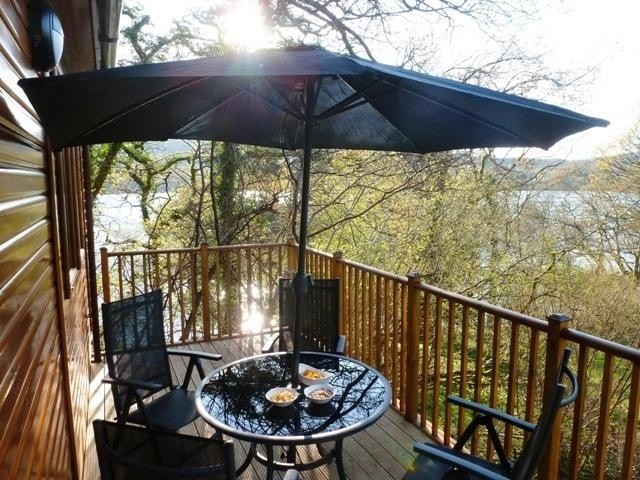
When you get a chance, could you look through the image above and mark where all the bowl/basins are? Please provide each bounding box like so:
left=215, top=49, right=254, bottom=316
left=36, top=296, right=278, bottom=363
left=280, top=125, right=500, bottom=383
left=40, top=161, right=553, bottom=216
left=303, top=384, right=337, bottom=405
left=298, top=363, right=336, bottom=387
left=265, top=387, right=299, bottom=408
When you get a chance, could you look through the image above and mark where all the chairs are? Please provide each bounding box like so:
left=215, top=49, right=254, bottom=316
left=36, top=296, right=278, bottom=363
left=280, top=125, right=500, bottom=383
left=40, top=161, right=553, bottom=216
left=401, top=347, right=579, bottom=480
left=93, top=288, right=239, bottom=479
left=262, top=275, right=346, bottom=357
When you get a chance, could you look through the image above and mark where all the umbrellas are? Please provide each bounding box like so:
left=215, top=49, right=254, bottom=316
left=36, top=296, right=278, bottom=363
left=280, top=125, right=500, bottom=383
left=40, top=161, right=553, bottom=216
left=17, top=42, right=609, bottom=465
